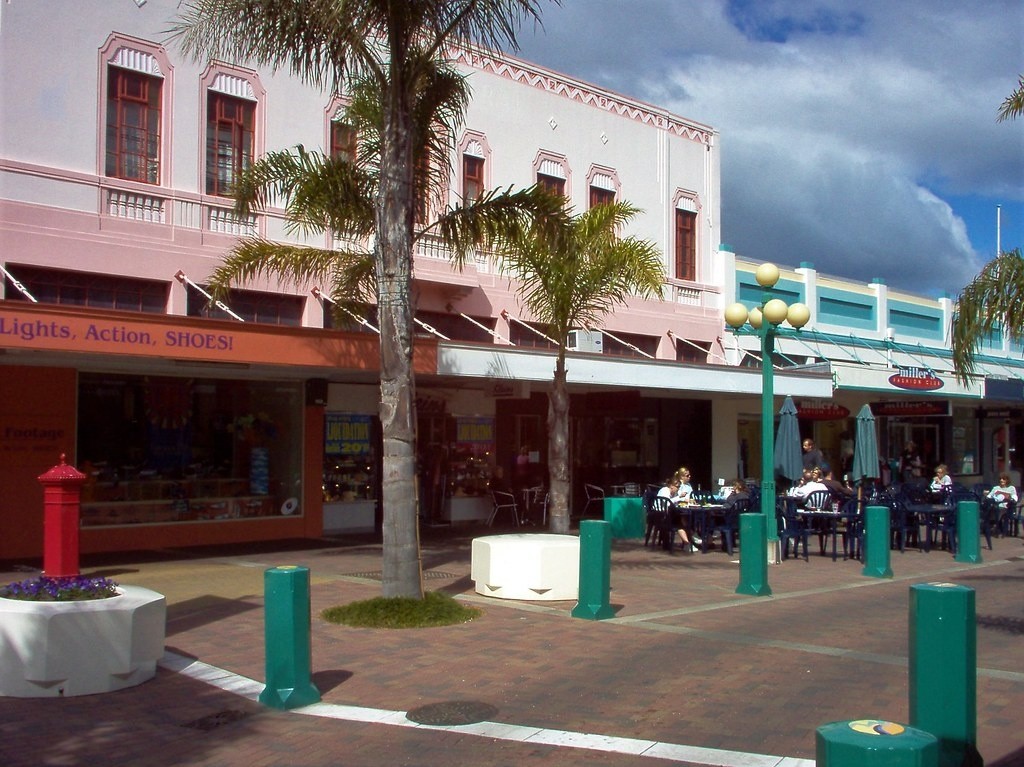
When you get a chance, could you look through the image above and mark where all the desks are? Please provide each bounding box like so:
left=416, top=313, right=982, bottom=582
left=907, top=502, right=954, bottom=552
left=781, top=494, right=804, bottom=535
left=794, top=507, right=854, bottom=562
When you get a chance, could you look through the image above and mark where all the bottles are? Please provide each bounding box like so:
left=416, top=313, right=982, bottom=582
left=697, top=484, right=703, bottom=504
left=703, top=495, right=707, bottom=505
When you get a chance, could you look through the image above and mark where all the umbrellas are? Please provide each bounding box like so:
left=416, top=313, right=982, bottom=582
left=852, top=403, right=880, bottom=515
left=774, top=394, right=803, bottom=486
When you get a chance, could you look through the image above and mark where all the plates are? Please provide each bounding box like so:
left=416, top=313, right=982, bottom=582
left=711, top=505, right=723, bottom=507
left=689, top=504, right=701, bottom=506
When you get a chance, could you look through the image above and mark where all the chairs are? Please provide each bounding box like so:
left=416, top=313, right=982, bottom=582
left=473, top=456, right=1024, bottom=566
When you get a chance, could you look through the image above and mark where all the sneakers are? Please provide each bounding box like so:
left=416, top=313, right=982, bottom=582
left=691, top=533, right=702, bottom=544
left=684, top=545, right=699, bottom=552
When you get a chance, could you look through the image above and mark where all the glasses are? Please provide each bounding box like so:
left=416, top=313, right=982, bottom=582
left=675, top=485, right=681, bottom=488
left=684, top=473, right=691, bottom=477
left=813, top=472, right=818, bottom=474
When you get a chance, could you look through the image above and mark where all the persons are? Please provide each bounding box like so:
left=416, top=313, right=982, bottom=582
left=674, top=467, right=693, bottom=503
left=930, top=464, right=952, bottom=521
left=898, top=440, right=920, bottom=480
left=490, top=466, right=525, bottom=523
left=985, top=472, right=1018, bottom=538
left=653, top=477, right=702, bottom=551
left=785, top=440, right=853, bottom=529
left=723, top=479, right=753, bottom=510
left=904, top=469, right=932, bottom=494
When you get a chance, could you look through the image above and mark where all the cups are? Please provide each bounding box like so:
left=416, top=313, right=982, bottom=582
left=832, top=503, right=839, bottom=514
left=690, top=499, right=696, bottom=505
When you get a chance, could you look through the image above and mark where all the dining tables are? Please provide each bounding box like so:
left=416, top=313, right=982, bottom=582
left=677, top=498, right=727, bottom=556
